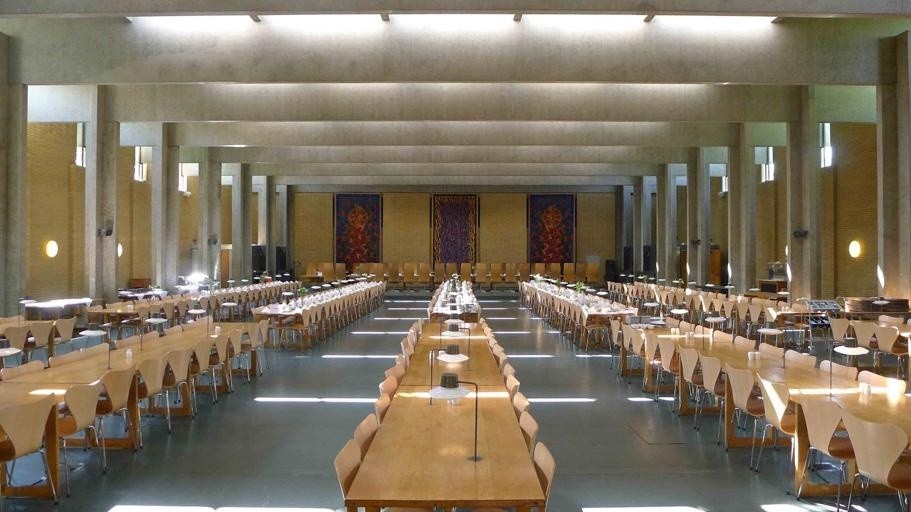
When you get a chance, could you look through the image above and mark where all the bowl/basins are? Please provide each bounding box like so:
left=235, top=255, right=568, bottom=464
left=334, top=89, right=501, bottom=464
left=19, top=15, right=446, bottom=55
left=632, top=323, right=654, bottom=330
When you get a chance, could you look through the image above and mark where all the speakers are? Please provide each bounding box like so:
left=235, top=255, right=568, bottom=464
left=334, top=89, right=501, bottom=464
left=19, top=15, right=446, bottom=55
left=605, top=259, right=617, bottom=281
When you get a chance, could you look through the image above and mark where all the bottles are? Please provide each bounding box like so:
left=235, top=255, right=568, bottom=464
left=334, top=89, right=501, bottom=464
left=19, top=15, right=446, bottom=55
left=747, top=352, right=760, bottom=361
left=215, top=326, right=220, bottom=333
left=670, top=327, right=679, bottom=334
left=781, top=305, right=791, bottom=312
left=685, top=332, right=694, bottom=340
left=865, top=385, right=872, bottom=397
left=127, top=349, right=132, bottom=358
left=738, top=293, right=744, bottom=304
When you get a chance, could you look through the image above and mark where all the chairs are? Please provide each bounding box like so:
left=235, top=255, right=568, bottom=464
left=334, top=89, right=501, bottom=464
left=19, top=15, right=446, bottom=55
left=2, top=262, right=388, bottom=510
left=333, top=263, right=558, bottom=511
left=518, top=263, right=911, bottom=512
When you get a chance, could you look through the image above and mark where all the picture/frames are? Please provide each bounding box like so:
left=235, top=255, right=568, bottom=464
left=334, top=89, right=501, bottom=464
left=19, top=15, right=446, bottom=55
left=429, top=192, right=478, bottom=267
left=528, top=191, right=577, bottom=265
left=333, top=191, right=382, bottom=268
